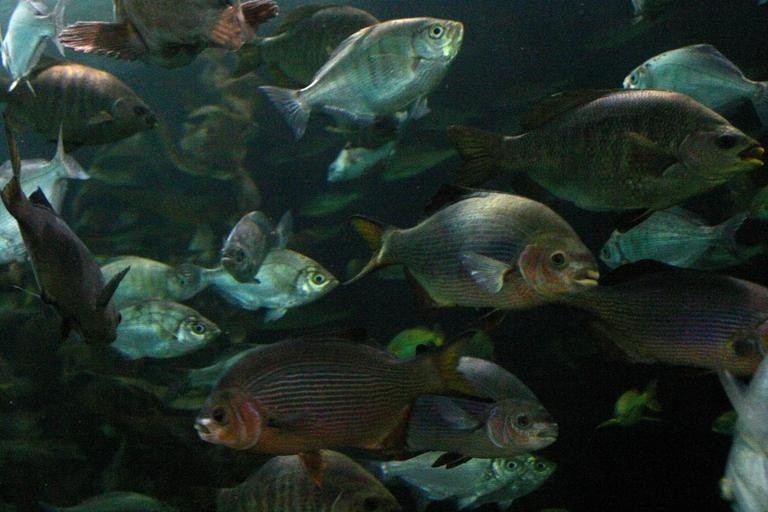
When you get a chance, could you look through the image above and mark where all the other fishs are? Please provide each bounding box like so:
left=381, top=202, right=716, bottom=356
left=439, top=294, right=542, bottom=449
left=0, top=1, right=560, bottom=512
